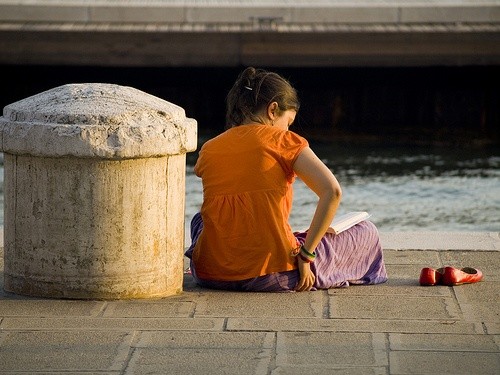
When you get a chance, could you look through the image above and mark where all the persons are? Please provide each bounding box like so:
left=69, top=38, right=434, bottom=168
left=184, top=66, right=389, bottom=293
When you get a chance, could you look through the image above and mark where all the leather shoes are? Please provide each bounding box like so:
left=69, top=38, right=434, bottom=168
left=419, top=265, right=483, bottom=287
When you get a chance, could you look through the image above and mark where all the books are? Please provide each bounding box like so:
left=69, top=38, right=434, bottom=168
left=293, top=211, right=372, bottom=235
